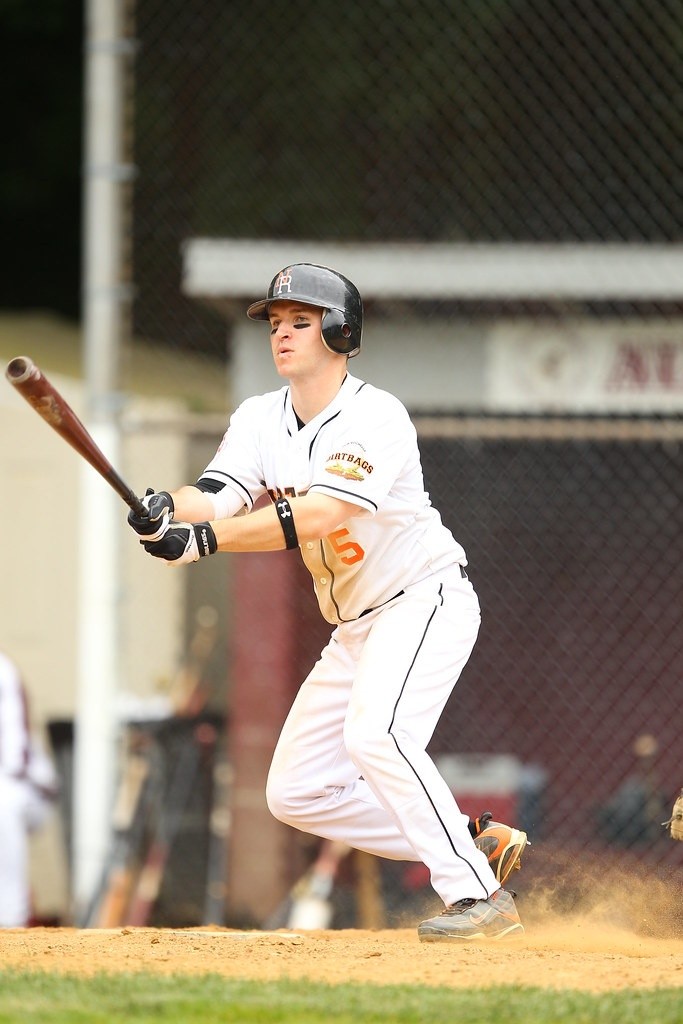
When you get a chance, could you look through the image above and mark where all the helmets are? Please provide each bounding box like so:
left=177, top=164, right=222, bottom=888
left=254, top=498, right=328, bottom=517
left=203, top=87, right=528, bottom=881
left=246, top=263, right=363, bottom=358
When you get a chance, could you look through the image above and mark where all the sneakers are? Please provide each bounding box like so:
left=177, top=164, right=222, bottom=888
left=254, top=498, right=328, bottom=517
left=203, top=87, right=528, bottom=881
left=418, top=887, right=525, bottom=943
left=472, top=810, right=532, bottom=885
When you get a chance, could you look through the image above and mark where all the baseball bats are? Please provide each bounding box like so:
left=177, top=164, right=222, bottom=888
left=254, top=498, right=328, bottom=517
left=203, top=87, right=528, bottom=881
left=1, top=353, right=151, bottom=519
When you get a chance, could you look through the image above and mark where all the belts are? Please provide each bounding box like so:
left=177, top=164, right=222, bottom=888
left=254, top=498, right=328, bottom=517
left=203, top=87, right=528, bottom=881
left=354, top=561, right=468, bottom=621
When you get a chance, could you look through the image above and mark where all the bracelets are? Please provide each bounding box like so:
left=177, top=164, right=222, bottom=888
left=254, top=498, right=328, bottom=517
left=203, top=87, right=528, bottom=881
left=275, top=498, right=298, bottom=550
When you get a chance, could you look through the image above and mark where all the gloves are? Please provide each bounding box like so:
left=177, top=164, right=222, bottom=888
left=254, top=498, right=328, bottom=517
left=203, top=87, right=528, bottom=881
left=127, top=488, right=174, bottom=541
left=140, top=519, right=218, bottom=568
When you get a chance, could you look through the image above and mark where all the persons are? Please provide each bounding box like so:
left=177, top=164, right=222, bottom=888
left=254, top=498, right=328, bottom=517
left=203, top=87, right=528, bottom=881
left=128, top=263, right=527, bottom=943
